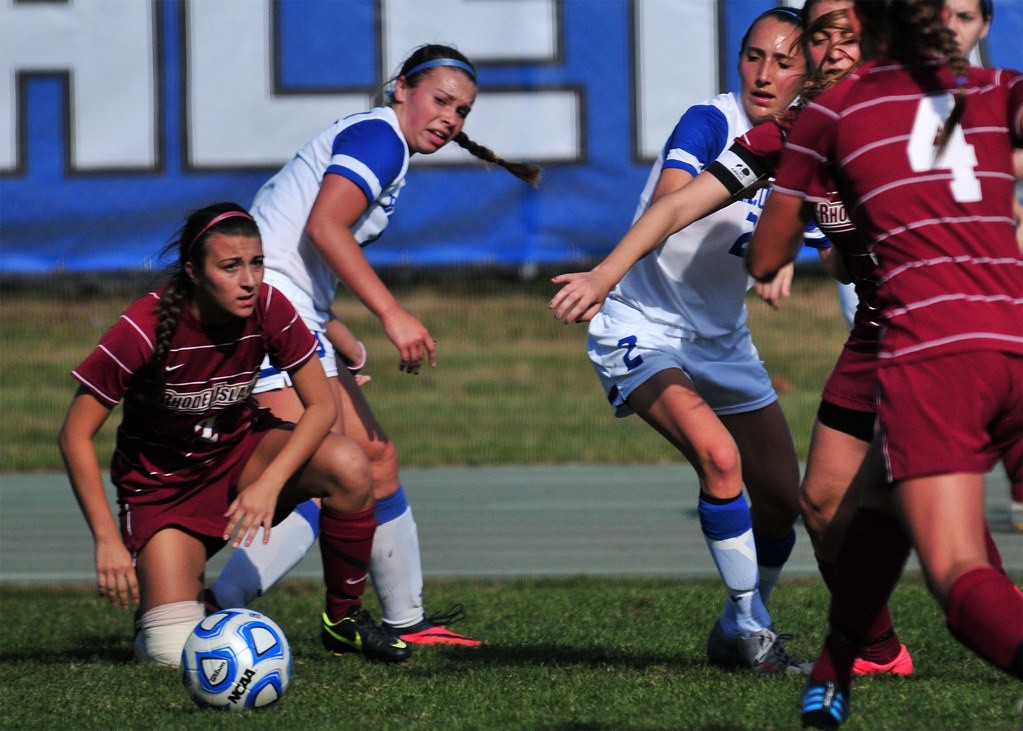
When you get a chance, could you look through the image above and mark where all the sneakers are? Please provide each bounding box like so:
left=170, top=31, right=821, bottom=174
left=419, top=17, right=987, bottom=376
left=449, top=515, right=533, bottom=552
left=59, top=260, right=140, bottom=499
left=709, top=618, right=808, bottom=680
left=802, top=679, right=850, bottom=731
left=382, top=605, right=483, bottom=648
left=318, top=613, right=411, bottom=662
left=850, top=644, right=913, bottom=677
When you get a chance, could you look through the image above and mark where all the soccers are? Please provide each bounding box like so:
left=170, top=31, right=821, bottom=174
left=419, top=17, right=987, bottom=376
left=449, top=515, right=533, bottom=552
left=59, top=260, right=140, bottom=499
left=180, top=607, right=293, bottom=714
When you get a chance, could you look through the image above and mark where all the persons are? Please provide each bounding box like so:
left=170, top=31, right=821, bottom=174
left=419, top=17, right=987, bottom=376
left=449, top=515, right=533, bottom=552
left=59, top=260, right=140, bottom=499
left=935, top=0, right=1023, bottom=532
left=548, top=0, right=915, bottom=731
left=59, top=202, right=411, bottom=671
left=204, top=43, right=541, bottom=646
left=586, top=8, right=814, bottom=677
left=744, top=0, right=1023, bottom=690
